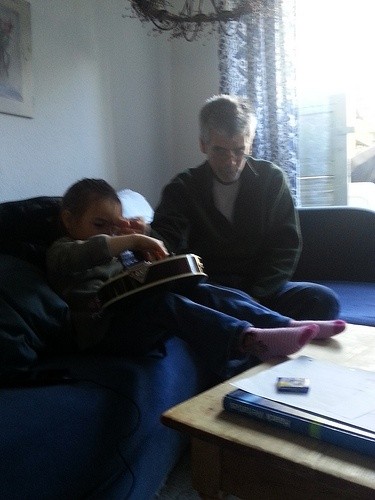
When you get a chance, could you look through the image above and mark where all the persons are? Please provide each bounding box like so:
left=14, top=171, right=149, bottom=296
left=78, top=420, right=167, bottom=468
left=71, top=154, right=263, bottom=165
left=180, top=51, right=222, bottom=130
left=150, top=94, right=340, bottom=380
left=44, top=179, right=346, bottom=362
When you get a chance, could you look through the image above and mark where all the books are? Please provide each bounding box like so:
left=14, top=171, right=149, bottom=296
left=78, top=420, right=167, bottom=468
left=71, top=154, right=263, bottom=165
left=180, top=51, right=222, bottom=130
left=223, top=355, right=375, bottom=458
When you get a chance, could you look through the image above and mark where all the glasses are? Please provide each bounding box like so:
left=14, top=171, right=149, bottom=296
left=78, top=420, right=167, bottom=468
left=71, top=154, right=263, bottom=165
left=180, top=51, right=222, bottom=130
left=203, top=139, right=250, bottom=160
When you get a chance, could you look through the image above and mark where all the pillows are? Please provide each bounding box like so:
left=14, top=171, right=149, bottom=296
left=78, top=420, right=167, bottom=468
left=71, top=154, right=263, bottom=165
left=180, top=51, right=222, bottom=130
left=0, top=252, right=70, bottom=364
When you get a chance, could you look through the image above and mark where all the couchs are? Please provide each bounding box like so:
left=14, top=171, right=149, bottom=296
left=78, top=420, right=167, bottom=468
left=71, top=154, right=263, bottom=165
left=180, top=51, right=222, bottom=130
left=0, top=196, right=211, bottom=500
left=292, top=211, right=375, bottom=327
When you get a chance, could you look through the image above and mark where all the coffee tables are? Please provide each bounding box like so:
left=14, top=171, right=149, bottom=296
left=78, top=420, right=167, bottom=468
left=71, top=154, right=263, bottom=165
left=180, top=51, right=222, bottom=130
left=160, top=324, right=375, bottom=500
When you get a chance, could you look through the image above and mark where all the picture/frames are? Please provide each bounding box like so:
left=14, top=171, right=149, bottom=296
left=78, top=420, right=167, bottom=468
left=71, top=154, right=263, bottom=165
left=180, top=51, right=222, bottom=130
left=0, top=0, right=36, bottom=119
